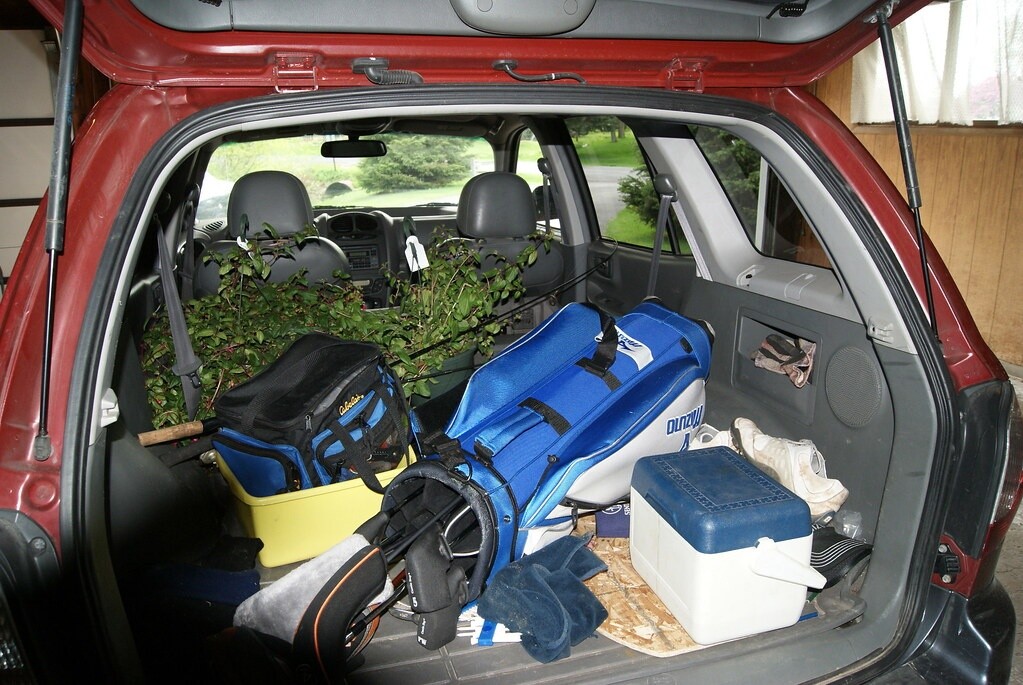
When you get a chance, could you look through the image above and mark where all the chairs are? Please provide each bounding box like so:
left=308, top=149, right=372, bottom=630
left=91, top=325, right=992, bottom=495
left=196, top=171, right=350, bottom=305
left=430, top=170, right=564, bottom=307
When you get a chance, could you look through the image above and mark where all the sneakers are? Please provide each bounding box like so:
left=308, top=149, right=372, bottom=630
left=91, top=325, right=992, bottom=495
left=686, top=422, right=739, bottom=454
left=730, top=416, right=849, bottom=526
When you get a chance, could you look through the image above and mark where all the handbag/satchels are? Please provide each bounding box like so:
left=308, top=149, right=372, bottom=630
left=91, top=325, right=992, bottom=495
left=212, top=331, right=415, bottom=497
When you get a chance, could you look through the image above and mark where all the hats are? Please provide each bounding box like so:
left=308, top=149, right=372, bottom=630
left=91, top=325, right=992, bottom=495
left=807, top=525, right=873, bottom=592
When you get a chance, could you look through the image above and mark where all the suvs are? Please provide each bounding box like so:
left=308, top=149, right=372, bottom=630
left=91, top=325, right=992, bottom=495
left=0, top=0, right=1023, bottom=685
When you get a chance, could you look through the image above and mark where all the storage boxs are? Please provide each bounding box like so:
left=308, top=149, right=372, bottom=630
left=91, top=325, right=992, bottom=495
left=214, top=445, right=418, bottom=571
left=630, top=445, right=826, bottom=646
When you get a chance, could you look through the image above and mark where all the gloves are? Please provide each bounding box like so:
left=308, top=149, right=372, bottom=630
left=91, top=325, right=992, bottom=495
left=755, top=334, right=817, bottom=389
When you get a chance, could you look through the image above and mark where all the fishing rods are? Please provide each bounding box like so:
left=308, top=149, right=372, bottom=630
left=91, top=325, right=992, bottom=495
left=134, top=235, right=619, bottom=449
